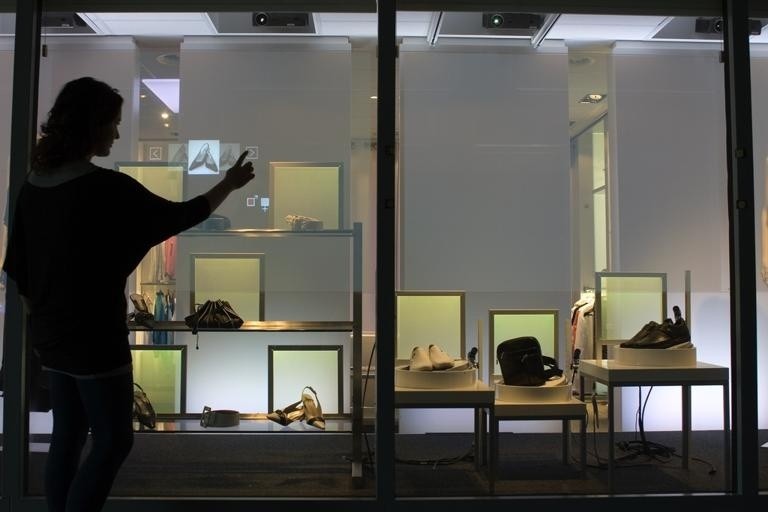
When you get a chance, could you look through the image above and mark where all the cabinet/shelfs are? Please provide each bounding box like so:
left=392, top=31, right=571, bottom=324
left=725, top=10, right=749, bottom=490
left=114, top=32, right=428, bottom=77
left=127, top=223, right=363, bottom=486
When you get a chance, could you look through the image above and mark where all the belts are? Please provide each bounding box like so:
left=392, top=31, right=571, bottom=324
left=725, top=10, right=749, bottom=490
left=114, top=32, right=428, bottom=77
left=200, top=406, right=240, bottom=429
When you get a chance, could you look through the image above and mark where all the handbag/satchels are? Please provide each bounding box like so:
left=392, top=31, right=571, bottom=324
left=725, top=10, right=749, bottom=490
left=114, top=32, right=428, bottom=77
left=184, top=299, right=244, bottom=328
left=284, top=214, right=324, bottom=231
left=496, top=336, right=563, bottom=386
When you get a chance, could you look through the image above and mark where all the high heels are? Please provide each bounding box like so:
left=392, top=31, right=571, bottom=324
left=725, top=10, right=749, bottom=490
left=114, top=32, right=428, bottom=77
left=189, top=143, right=217, bottom=172
left=129, top=292, right=156, bottom=328
left=266, top=386, right=326, bottom=430
left=133, top=383, right=157, bottom=429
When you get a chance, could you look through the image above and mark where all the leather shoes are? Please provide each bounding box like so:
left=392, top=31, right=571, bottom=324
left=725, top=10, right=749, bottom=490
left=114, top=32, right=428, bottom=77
left=409, top=343, right=455, bottom=372
left=620, top=317, right=691, bottom=350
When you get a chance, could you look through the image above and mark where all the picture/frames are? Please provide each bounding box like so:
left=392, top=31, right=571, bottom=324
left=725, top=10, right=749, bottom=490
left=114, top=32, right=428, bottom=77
left=130, top=344, right=187, bottom=418
left=269, top=162, right=344, bottom=230
left=268, top=344, right=343, bottom=417
left=489, top=309, right=560, bottom=381
left=596, top=273, right=667, bottom=345
left=189, top=252, right=265, bottom=321
left=396, top=290, right=466, bottom=364
left=116, top=161, right=184, bottom=204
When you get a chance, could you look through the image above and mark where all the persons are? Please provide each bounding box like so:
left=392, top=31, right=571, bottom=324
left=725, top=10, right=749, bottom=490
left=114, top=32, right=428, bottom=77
left=1, top=77, right=255, bottom=512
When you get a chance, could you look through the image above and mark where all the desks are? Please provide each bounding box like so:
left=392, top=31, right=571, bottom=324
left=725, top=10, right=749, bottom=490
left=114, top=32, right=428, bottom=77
left=479, top=400, right=587, bottom=473
left=579, top=359, right=731, bottom=495
left=395, top=380, right=494, bottom=496
